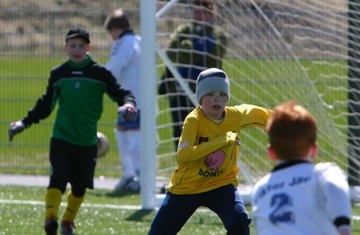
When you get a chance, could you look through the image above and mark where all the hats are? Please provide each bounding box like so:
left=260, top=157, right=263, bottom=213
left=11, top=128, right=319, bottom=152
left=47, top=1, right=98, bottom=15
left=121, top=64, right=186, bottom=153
left=197, top=69, right=232, bottom=104
left=66, top=28, right=91, bottom=43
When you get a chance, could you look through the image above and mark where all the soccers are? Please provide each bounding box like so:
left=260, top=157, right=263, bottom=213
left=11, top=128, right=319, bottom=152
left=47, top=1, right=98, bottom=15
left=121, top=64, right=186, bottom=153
left=95, top=129, right=110, bottom=157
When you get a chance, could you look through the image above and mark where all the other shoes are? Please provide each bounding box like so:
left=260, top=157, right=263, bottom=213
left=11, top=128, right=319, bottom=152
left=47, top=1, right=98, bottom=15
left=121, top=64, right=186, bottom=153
left=61, top=220, right=76, bottom=235
left=45, top=219, right=58, bottom=235
left=116, top=171, right=140, bottom=190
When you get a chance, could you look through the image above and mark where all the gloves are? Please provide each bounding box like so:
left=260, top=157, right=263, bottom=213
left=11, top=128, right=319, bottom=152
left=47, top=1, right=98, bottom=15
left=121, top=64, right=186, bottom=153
left=117, top=101, right=141, bottom=131
left=9, top=120, right=25, bottom=142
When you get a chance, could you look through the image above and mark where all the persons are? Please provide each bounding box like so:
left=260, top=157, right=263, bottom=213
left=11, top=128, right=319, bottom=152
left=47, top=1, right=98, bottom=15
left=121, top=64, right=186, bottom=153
left=9, top=28, right=139, bottom=235
left=158, top=0, right=227, bottom=193
left=252, top=103, right=351, bottom=235
left=148, top=68, right=273, bottom=235
left=104, top=11, right=140, bottom=191
